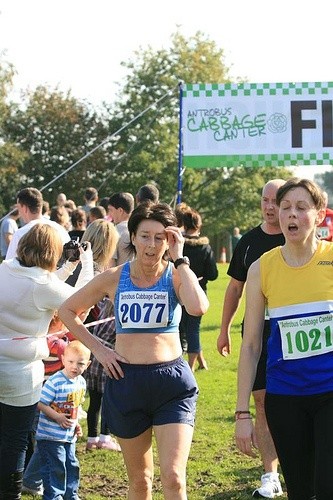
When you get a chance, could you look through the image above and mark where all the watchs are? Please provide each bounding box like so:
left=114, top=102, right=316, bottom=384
left=173, top=256, right=191, bottom=267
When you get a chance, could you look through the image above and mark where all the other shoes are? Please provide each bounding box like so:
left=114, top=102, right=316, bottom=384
left=87, top=442, right=98, bottom=449
left=98, top=438, right=121, bottom=451
left=22, top=486, right=43, bottom=495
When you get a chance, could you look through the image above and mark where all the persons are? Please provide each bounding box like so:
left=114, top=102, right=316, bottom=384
left=57, top=201, right=209, bottom=500
left=236, top=177, right=333, bottom=500
left=314, top=191, right=333, bottom=243
left=0, top=224, right=94, bottom=500
left=217, top=179, right=297, bottom=500
left=36, top=340, right=88, bottom=500
left=0, top=185, right=218, bottom=454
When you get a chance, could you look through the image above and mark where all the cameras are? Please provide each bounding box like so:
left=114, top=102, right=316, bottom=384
left=63, top=240, right=87, bottom=262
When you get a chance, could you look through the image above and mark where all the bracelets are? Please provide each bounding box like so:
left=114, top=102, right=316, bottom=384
left=235, top=410, right=251, bottom=414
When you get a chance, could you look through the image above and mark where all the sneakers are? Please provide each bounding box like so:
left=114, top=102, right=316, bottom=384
left=252, top=472, right=283, bottom=498
